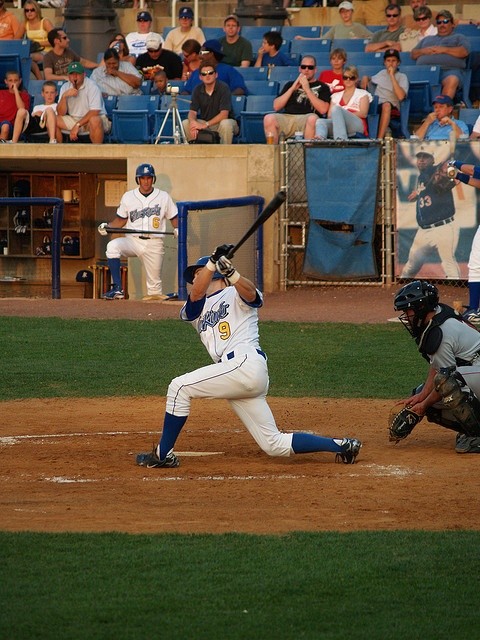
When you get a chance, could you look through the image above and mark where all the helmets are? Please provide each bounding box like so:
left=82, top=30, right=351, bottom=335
left=136, top=164, right=158, bottom=185
left=11, top=179, right=29, bottom=197
left=62, top=235, right=79, bottom=255
left=394, top=282, right=439, bottom=338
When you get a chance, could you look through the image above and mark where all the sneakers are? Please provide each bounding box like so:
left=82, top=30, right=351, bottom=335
left=455, top=434, right=480, bottom=453
left=462, top=309, right=480, bottom=326
left=2, top=137, right=15, bottom=145
left=137, top=447, right=181, bottom=469
left=48, top=139, right=56, bottom=144
left=100, top=289, right=125, bottom=301
left=340, top=437, right=362, bottom=465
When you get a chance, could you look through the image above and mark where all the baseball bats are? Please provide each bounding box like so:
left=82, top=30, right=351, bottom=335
left=99, top=227, right=175, bottom=236
left=225, top=191, right=287, bottom=261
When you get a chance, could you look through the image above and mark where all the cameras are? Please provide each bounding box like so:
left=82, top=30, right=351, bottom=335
left=166, top=83, right=181, bottom=98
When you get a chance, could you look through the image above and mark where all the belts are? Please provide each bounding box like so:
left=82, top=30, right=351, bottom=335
left=420, top=217, right=454, bottom=229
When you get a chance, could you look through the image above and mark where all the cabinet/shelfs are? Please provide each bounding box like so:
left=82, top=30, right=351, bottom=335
left=0, top=168, right=94, bottom=261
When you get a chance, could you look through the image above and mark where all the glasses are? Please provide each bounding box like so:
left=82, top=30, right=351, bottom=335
left=434, top=19, right=451, bottom=25
left=199, top=71, right=214, bottom=77
left=24, top=8, right=37, bottom=13
left=182, top=52, right=194, bottom=59
left=199, top=50, right=211, bottom=56
left=386, top=13, right=400, bottom=18
left=178, top=16, right=191, bottom=19
left=300, top=64, right=315, bottom=70
left=137, top=19, right=152, bottom=22
left=415, top=16, right=428, bottom=22
left=114, top=37, right=125, bottom=41
left=147, top=47, right=161, bottom=53
left=341, top=74, right=356, bottom=83
left=58, top=33, right=69, bottom=41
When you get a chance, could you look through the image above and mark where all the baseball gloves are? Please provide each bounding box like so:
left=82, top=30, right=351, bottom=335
left=387, top=401, right=423, bottom=445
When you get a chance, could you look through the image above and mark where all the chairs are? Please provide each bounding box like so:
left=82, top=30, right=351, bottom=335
left=1, top=22, right=480, bottom=145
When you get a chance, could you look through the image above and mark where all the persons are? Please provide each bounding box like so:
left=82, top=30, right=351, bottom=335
left=181, top=60, right=240, bottom=144
left=469, top=114, right=480, bottom=139
left=388, top=278, right=480, bottom=453
left=0, top=71, right=29, bottom=143
left=163, top=7, right=209, bottom=63
left=401, top=0, right=480, bottom=30
left=181, top=39, right=217, bottom=81
left=365, top=4, right=405, bottom=52
left=12, top=0, right=56, bottom=80
left=413, top=95, right=469, bottom=140
left=43, top=28, right=99, bottom=81
left=89, top=48, right=145, bottom=96
left=219, top=15, right=253, bottom=68
left=99, top=33, right=136, bottom=66
left=312, top=66, right=373, bottom=140
left=97, top=163, right=179, bottom=300
left=136, top=255, right=362, bottom=468
left=412, top=10, right=472, bottom=99
left=295, top=1, right=375, bottom=40
left=252, top=31, right=295, bottom=66
left=125, top=11, right=165, bottom=58
left=447, top=157, right=480, bottom=323
left=0, top=81, right=58, bottom=144
left=400, top=146, right=462, bottom=279
left=46, top=62, right=112, bottom=144
left=263, top=55, right=330, bottom=144
left=151, top=71, right=181, bottom=95
left=318, top=48, right=347, bottom=94
left=412, top=6, right=438, bottom=52
left=134, top=34, right=184, bottom=80
left=0, top=0, right=20, bottom=40
left=361, top=49, right=409, bottom=139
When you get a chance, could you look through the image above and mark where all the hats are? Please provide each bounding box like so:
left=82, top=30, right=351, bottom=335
left=146, top=32, right=163, bottom=50
left=337, top=0, right=356, bottom=13
left=76, top=269, right=95, bottom=283
left=384, top=48, right=400, bottom=59
left=183, top=254, right=226, bottom=283
left=203, top=39, right=226, bottom=59
left=66, top=62, right=85, bottom=75
left=223, top=14, right=240, bottom=24
left=414, top=145, right=437, bottom=159
left=431, top=94, right=455, bottom=106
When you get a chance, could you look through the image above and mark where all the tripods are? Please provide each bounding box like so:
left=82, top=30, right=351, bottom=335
left=154, top=102, right=188, bottom=146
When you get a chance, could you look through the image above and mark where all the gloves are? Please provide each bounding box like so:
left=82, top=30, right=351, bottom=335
left=174, top=228, right=178, bottom=237
left=97, top=223, right=109, bottom=236
left=213, top=243, right=235, bottom=262
left=215, top=256, right=235, bottom=278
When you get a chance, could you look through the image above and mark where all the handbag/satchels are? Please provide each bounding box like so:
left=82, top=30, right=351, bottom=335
left=23, top=29, right=45, bottom=54
